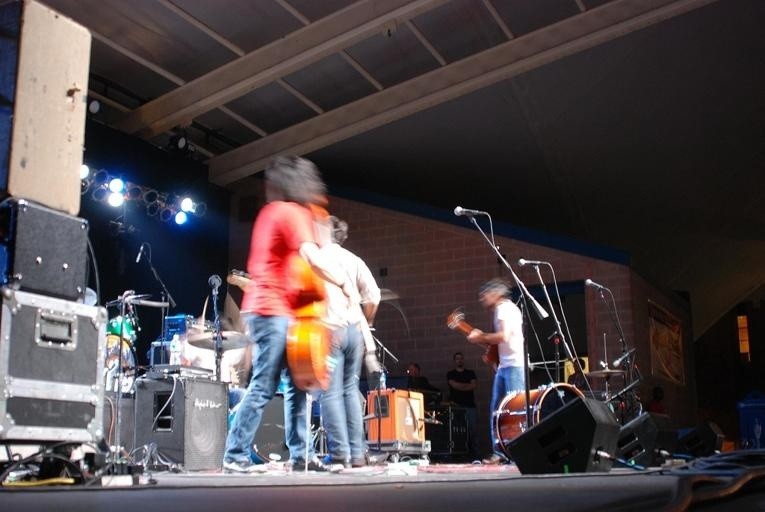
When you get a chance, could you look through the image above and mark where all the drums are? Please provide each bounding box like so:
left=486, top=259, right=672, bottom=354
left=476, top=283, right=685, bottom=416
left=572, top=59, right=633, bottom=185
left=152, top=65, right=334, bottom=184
left=492, top=381, right=585, bottom=461
left=104, top=334, right=139, bottom=393
left=106, top=313, right=138, bottom=345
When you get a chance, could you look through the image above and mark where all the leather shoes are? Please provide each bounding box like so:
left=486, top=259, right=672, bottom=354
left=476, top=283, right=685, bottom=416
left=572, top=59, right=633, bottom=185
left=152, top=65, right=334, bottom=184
left=483, top=454, right=508, bottom=464
left=323, top=456, right=365, bottom=467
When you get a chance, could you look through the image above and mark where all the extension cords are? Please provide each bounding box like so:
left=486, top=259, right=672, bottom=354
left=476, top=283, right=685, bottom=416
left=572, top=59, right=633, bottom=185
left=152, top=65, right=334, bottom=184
left=87, top=473, right=148, bottom=487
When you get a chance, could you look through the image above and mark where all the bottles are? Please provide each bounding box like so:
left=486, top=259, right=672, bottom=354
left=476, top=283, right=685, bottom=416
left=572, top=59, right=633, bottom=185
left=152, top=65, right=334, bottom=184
left=168, top=334, right=183, bottom=365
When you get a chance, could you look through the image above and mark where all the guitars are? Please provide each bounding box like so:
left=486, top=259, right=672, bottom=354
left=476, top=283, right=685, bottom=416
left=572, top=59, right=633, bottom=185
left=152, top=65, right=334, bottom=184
left=447, top=310, right=500, bottom=371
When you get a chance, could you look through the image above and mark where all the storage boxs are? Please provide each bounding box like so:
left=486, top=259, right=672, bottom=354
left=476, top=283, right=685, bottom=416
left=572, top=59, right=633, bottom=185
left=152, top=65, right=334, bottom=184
left=2, top=287, right=107, bottom=447
left=1, top=197, right=89, bottom=301
left=0, top=1, right=92, bottom=216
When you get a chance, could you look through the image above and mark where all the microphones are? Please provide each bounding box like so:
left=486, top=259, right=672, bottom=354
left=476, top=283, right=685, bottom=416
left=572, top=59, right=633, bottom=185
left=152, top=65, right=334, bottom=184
left=135, top=246, right=144, bottom=263
left=420, top=418, right=444, bottom=426
left=613, top=348, right=639, bottom=367
left=128, top=304, right=142, bottom=332
left=517, top=258, right=548, bottom=267
left=585, top=279, right=609, bottom=292
left=454, top=206, right=488, bottom=216
left=208, top=275, right=222, bottom=288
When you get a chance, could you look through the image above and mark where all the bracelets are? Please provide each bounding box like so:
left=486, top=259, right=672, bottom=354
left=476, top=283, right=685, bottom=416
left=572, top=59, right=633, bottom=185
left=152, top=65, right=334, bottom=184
left=340, top=281, right=346, bottom=288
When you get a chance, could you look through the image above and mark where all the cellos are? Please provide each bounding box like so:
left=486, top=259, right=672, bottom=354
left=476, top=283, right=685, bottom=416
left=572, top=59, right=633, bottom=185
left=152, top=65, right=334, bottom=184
left=286, top=194, right=333, bottom=392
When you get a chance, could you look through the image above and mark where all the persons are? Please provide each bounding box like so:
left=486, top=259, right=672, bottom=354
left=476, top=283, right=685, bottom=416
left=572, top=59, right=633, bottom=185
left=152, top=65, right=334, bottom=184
left=465, top=279, right=528, bottom=466
left=407, top=363, right=436, bottom=390
left=446, top=352, right=477, bottom=463
left=222, top=153, right=355, bottom=474
left=320, top=215, right=381, bottom=467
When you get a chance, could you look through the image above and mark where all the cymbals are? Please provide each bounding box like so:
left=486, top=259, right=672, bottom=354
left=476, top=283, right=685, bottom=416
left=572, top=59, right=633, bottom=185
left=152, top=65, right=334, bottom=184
left=188, top=330, right=250, bottom=350
left=587, top=369, right=624, bottom=378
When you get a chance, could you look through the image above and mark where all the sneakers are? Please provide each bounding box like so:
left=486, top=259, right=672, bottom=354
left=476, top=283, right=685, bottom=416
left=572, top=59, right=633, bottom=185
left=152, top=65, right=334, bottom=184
left=224, top=461, right=267, bottom=472
left=292, top=461, right=332, bottom=471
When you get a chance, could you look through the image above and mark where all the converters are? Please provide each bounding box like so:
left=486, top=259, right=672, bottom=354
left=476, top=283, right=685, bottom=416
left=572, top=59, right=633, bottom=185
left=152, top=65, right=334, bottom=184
left=127, top=465, right=144, bottom=476
left=83, top=452, right=106, bottom=468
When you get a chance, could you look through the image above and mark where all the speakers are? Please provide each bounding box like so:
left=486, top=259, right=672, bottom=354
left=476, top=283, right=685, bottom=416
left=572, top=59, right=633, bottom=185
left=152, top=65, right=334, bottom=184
left=611, top=411, right=674, bottom=468
left=670, top=426, right=717, bottom=458
left=506, top=396, right=621, bottom=475
left=367, top=389, right=426, bottom=443
left=103, top=392, right=135, bottom=456
left=135, top=377, right=229, bottom=473
